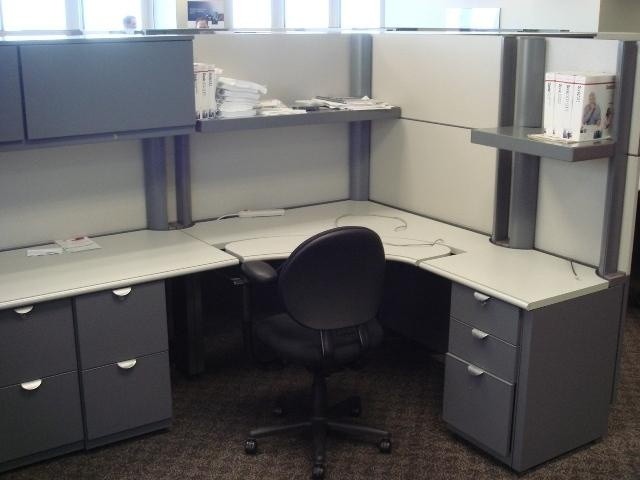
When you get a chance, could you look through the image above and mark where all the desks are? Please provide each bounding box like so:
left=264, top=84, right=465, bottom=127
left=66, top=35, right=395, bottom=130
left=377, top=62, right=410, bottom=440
left=180, top=198, right=628, bottom=471
left=1, top=228, right=239, bottom=471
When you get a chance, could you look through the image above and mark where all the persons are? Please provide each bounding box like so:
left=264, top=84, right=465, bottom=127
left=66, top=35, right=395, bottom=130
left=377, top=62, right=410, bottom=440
left=123, top=16, right=137, bottom=30
left=196, top=17, right=209, bottom=29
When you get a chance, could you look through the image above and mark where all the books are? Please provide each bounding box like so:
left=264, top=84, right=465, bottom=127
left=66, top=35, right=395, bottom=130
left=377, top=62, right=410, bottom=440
left=194, top=63, right=395, bottom=120
left=544, top=72, right=616, bottom=142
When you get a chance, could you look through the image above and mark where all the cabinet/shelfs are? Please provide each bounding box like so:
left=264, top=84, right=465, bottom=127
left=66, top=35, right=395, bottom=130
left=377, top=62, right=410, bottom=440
left=0, top=40, right=26, bottom=152
left=20, top=35, right=196, bottom=150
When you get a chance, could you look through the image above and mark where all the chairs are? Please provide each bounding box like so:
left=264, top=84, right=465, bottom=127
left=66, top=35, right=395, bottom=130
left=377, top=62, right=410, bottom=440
left=240, top=227, right=390, bottom=479
